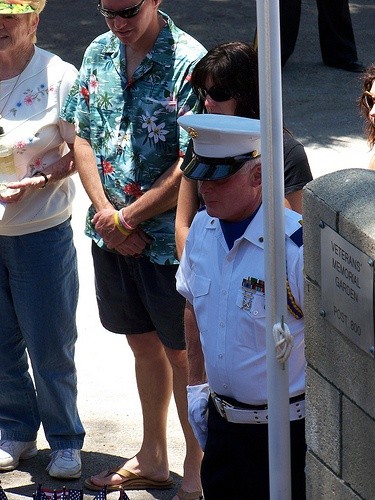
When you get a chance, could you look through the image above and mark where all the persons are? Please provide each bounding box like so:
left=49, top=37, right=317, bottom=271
left=252, top=0, right=366, bottom=72
left=0, top=0, right=84, bottom=478
left=60, top=0, right=207, bottom=500
left=174, top=42, right=313, bottom=259
left=359, top=63, right=375, bottom=171
left=173, top=113, right=307, bottom=500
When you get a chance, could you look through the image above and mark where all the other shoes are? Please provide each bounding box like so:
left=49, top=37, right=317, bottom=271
left=322, top=58, right=364, bottom=72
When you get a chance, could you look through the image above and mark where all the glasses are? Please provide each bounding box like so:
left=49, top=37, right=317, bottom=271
left=96, top=0, right=144, bottom=19
left=197, top=83, right=240, bottom=103
left=363, top=90, right=375, bottom=111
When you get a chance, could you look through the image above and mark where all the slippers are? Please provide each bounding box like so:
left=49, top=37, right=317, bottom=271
left=84, top=466, right=175, bottom=489
left=168, top=488, right=203, bottom=500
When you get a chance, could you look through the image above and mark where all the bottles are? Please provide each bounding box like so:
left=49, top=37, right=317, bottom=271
left=0, top=126, right=21, bottom=198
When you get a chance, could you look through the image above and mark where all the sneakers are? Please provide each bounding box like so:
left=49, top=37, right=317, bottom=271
left=45, top=447, right=81, bottom=478
left=0, top=440, right=37, bottom=471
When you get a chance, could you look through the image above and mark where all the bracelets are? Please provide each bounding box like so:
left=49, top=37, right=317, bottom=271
left=113, top=208, right=135, bottom=235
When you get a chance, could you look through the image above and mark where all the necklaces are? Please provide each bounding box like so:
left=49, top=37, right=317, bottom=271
left=0, top=47, right=33, bottom=135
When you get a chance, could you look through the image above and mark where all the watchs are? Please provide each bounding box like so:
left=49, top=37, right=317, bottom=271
left=33, top=171, right=49, bottom=189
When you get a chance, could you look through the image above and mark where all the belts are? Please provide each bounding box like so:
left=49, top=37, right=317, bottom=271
left=210, top=388, right=306, bottom=424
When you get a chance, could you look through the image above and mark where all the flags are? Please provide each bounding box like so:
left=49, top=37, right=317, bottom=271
left=33, top=484, right=130, bottom=500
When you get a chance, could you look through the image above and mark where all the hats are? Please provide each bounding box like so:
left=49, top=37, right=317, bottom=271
left=0, top=0, right=38, bottom=14
left=177, top=112, right=260, bottom=182
